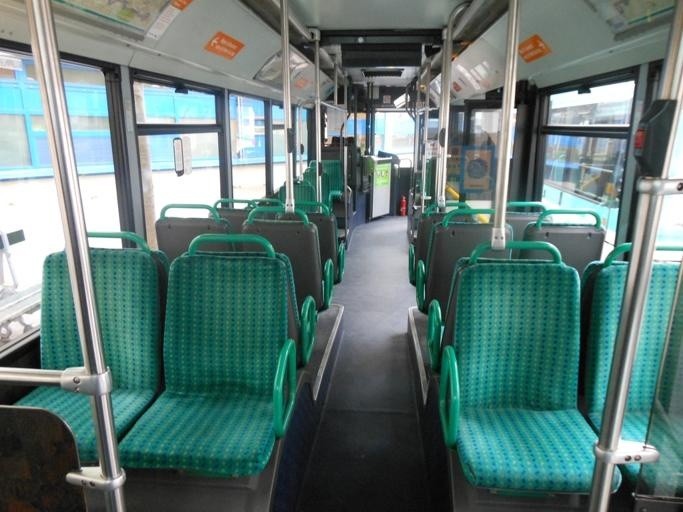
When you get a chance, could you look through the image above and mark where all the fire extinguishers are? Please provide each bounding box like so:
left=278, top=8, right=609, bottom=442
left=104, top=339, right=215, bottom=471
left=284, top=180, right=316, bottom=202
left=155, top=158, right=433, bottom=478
left=400, top=195, right=407, bottom=216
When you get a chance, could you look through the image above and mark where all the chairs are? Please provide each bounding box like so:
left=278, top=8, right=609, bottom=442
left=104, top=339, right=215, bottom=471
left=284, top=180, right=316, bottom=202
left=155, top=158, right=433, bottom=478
left=332, top=136, right=354, bottom=146
